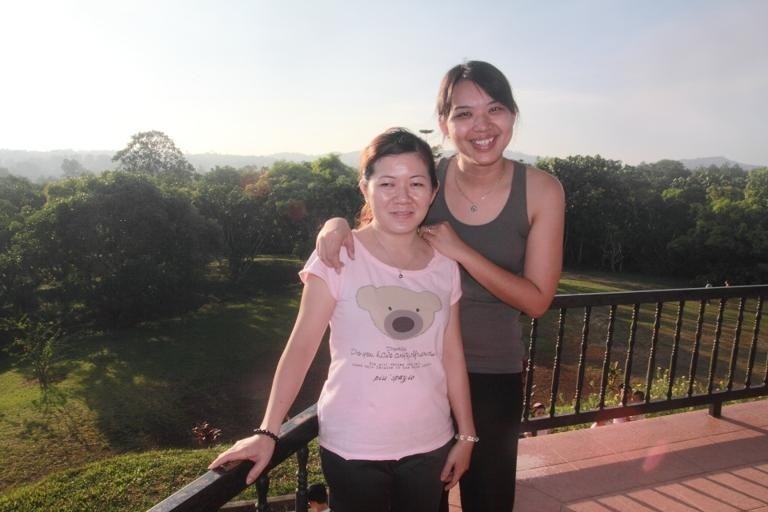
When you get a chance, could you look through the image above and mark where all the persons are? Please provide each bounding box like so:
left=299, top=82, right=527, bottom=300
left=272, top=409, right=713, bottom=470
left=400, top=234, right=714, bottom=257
left=705, top=279, right=730, bottom=306
left=590, top=383, right=645, bottom=428
